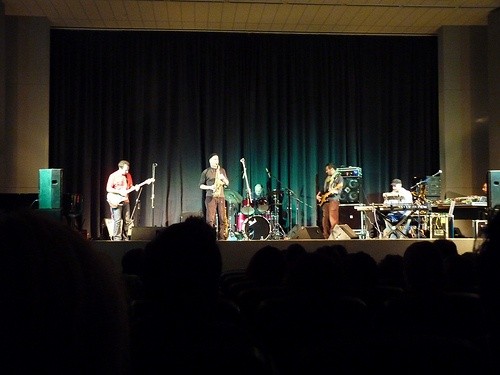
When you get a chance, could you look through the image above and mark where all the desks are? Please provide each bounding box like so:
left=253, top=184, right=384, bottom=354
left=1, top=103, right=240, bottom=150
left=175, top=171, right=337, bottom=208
left=431, top=206, right=487, bottom=238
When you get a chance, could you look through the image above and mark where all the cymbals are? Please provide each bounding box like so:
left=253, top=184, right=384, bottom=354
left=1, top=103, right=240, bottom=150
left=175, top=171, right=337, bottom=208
left=267, top=189, right=283, bottom=206
left=223, top=188, right=243, bottom=204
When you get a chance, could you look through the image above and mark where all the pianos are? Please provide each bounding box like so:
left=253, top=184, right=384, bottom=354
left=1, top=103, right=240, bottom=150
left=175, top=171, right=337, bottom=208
left=354, top=204, right=431, bottom=238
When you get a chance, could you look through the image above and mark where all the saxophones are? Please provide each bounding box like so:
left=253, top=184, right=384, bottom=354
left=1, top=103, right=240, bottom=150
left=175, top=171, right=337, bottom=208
left=212, top=164, right=225, bottom=198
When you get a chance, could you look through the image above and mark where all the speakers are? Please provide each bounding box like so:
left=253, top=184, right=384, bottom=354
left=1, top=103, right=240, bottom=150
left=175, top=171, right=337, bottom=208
left=131, top=227, right=162, bottom=240
left=328, top=223, right=359, bottom=239
left=293, top=226, right=325, bottom=240
left=339, top=177, right=363, bottom=204
left=487, top=170, right=500, bottom=209
left=38, top=168, right=63, bottom=209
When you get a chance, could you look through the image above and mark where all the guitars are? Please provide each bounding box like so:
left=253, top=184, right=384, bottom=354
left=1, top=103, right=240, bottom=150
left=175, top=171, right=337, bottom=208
left=107, top=178, right=156, bottom=209
left=318, top=182, right=344, bottom=207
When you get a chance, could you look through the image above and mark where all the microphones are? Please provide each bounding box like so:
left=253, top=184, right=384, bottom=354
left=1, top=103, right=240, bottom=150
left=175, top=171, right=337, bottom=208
left=266, top=168, right=271, bottom=178
left=432, top=169, right=442, bottom=177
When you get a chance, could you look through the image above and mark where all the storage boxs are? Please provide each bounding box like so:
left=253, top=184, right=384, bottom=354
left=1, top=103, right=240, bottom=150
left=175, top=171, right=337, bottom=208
left=472, top=220, right=488, bottom=238
left=339, top=203, right=363, bottom=232
left=430, top=215, right=454, bottom=238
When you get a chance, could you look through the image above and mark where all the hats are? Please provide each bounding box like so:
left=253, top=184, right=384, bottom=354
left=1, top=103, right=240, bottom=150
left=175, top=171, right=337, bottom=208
left=391, top=179, right=402, bottom=185
left=208, top=153, right=220, bottom=163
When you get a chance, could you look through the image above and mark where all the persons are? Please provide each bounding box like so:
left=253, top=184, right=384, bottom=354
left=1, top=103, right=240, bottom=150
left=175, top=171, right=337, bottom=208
left=199, top=152, right=229, bottom=240
left=316, top=163, right=343, bottom=239
left=251, top=184, right=266, bottom=200
left=107, top=161, right=140, bottom=241
left=383, top=179, right=413, bottom=239
left=1, top=210, right=500, bottom=375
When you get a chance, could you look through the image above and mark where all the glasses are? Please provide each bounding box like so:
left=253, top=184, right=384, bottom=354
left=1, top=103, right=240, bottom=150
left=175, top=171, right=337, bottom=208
left=392, top=185, right=396, bottom=187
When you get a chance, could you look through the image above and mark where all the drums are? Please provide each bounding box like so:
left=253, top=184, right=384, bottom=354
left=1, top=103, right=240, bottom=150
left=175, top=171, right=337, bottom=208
left=243, top=215, right=272, bottom=241
left=240, top=197, right=256, bottom=216
left=256, top=196, right=270, bottom=213
left=262, top=211, right=275, bottom=221
left=234, top=211, right=248, bottom=232
